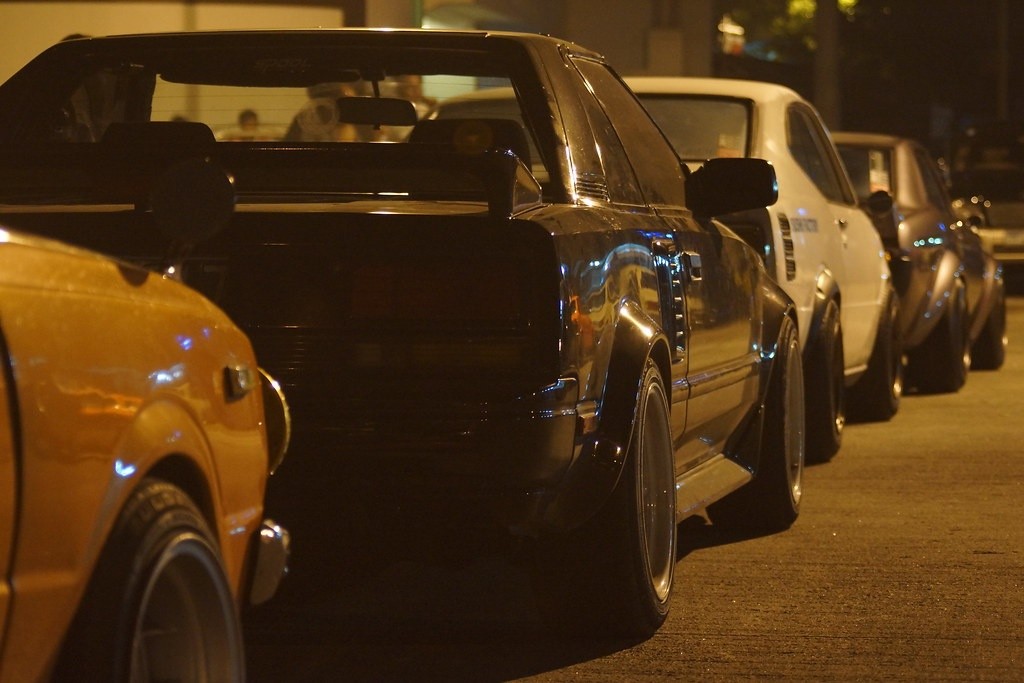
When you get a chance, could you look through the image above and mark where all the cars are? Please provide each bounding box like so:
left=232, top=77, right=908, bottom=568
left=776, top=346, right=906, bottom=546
left=945, top=160, right=1024, bottom=285
left=412, top=74, right=906, bottom=459
left=713, top=129, right=1008, bottom=392
left=0, top=26, right=811, bottom=635
left=0, top=117, right=298, bottom=683
